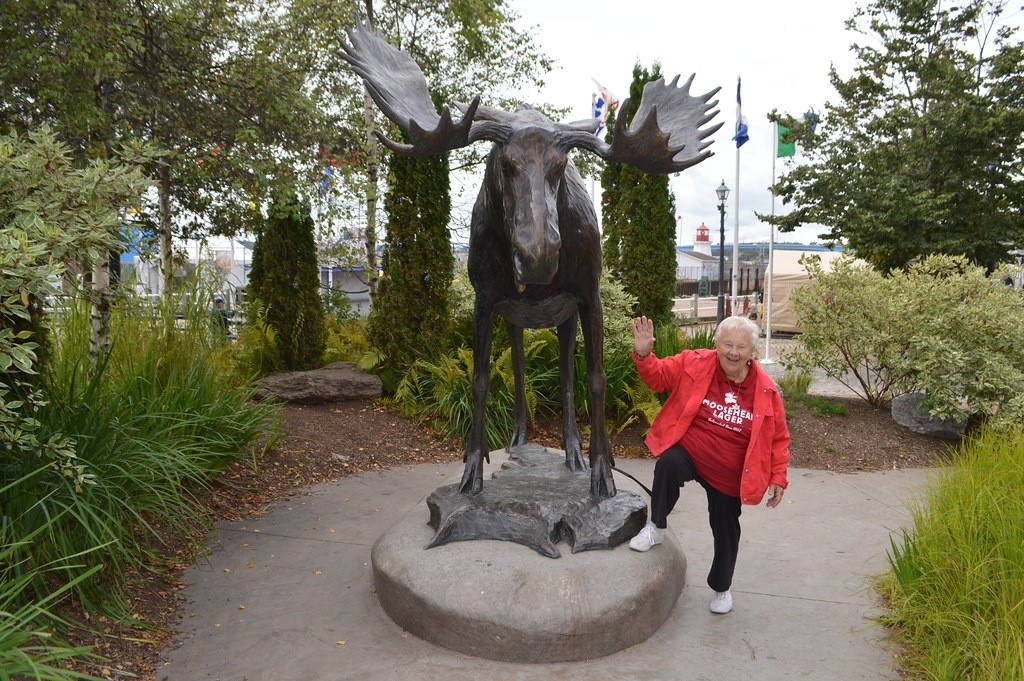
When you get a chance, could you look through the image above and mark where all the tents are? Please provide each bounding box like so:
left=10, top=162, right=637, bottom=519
left=762, top=250, right=1024, bottom=336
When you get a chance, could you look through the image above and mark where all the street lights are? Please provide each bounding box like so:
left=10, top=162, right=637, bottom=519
left=715, top=178, right=731, bottom=326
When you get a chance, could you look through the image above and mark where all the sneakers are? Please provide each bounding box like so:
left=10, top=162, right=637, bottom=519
left=629, top=522, right=663, bottom=550
left=710, top=590, right=732, bottom=612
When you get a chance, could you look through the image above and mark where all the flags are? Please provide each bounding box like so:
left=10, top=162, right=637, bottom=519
left=776, top=113, right=819, bottom=158
left=732, top=81, right=749, bottom=148
left=318, top=140, right=360, bottom=170
left=322, top=163, right=333, bottom=195
left=591, top=76, right=619, bottom=137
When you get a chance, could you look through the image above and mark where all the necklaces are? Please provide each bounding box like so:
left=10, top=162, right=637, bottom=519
left=727, top=376, right=740, bottom=408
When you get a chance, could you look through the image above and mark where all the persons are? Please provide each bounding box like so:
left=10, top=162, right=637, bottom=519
left=760, top=283, right=764, bottom=303
left=629, top=315, right=790, bottom=613
left=995, top=265, right=1013, bottom=288
left=210, top=297, right=239, bottom=346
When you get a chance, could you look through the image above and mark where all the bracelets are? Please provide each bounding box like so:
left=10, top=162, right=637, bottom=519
left=633, top=348, right=651, bottom=358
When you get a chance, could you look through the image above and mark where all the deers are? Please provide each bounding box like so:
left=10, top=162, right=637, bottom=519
left=334, top=8, right=726, bottom=497
